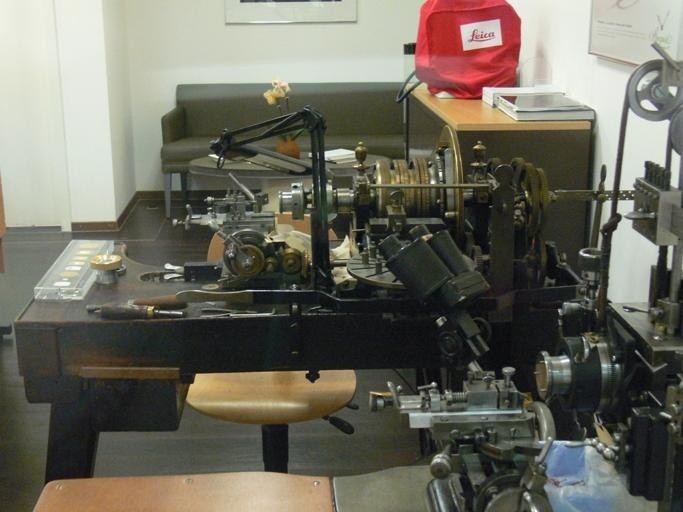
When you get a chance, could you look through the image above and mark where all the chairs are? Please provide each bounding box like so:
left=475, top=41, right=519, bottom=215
left=184, top=211, right=358, bottom=472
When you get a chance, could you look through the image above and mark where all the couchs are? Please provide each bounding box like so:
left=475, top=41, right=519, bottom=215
left=160, top=81, right=406, bottom=219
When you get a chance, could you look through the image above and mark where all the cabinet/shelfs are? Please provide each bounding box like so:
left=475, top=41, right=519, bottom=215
left=402, top=83, right=592, bottom=265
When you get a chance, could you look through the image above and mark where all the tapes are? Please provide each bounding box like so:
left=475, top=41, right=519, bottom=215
left=89, top=253, right=123, bottom=270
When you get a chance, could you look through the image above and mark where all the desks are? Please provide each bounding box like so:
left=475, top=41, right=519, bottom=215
left=11, top=237, right=587, bottom=487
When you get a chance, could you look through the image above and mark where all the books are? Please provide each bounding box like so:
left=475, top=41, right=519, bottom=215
left=309, top=147, right=357, bottom=164
left=482, top=85, right=595, bottom=122
